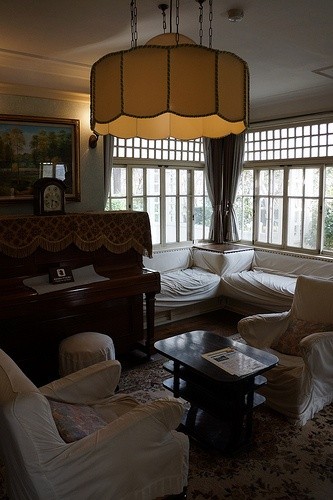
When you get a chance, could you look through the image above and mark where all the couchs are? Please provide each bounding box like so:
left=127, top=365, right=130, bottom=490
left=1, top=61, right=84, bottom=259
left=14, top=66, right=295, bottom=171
left=0, top=349, right=190, bottom=500
left=143, top=239, right=333, bottom=331
left=238, top=273, right=333, bottom=427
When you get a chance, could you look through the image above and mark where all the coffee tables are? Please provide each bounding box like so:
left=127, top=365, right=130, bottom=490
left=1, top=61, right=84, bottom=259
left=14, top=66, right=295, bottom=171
left=154, top=330, right=279, bottom=457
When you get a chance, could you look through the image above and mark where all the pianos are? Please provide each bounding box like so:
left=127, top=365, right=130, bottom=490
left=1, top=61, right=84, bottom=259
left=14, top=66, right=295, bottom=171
left=0, top=209, right=162, bottom=356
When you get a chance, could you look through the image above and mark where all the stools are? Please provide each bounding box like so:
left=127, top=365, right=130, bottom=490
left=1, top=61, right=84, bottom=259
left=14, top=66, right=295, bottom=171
left=60, top=331, right=119, bottom=392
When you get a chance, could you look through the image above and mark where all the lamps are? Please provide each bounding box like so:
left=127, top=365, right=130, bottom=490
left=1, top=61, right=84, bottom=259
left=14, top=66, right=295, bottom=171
left=89, top=0, right=251, bottom=141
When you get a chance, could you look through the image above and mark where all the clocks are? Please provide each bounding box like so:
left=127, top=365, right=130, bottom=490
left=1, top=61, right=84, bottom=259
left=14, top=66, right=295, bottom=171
left=33, top=176, right=67, bottom=215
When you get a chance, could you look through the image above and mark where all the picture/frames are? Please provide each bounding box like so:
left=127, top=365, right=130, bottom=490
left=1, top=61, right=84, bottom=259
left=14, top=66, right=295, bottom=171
left=0, top=114, right=81, bottom=207
